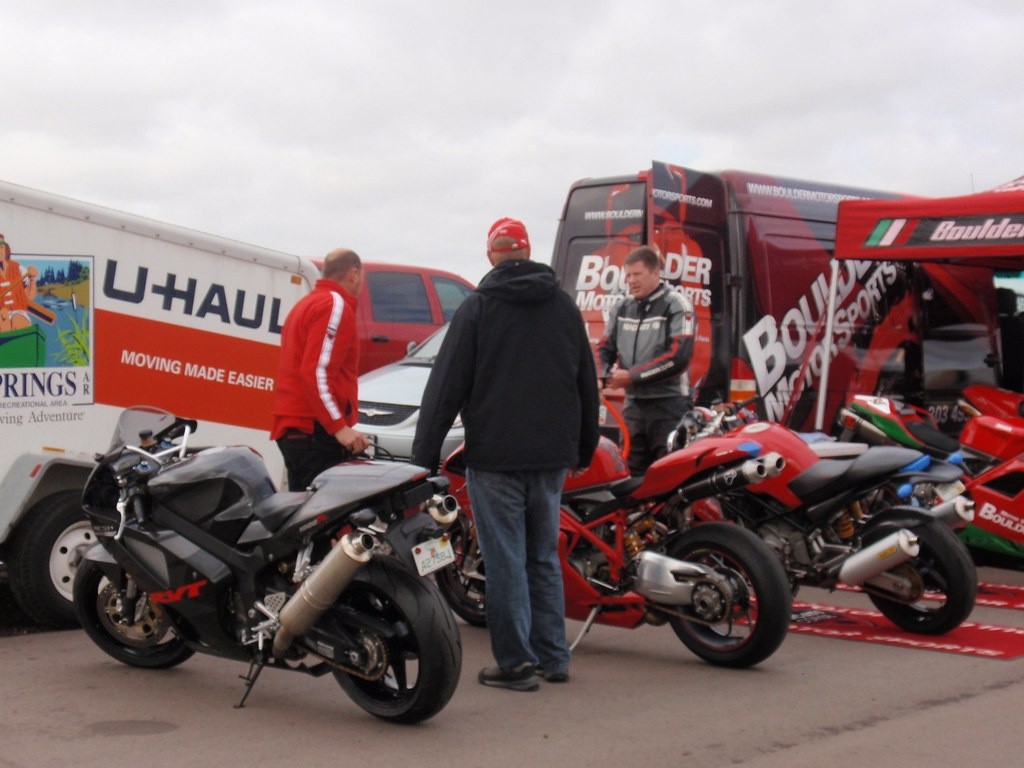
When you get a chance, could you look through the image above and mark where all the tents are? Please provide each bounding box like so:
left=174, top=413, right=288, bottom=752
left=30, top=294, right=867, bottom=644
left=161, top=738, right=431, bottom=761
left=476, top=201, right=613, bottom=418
left=814, top=174, right=1024, bottom=432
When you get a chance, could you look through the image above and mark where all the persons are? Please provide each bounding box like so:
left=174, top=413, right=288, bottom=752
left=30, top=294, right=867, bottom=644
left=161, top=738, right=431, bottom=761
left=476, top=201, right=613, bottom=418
left=270, top=247, right=369, bottom=493
left=0, top=234, right=38, bottom=332
left=593, top=246, right=695, bottom=479
left=409, top=218, right=600, bottom=692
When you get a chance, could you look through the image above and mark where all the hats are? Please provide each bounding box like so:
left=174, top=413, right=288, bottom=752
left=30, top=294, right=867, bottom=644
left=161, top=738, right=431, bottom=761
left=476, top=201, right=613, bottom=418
left=487, top=217, right=530, bottom=253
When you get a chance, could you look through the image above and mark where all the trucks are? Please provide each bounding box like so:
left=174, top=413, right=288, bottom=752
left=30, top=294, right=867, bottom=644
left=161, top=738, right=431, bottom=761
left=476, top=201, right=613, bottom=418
left=547, top=159, right=930, bottom=440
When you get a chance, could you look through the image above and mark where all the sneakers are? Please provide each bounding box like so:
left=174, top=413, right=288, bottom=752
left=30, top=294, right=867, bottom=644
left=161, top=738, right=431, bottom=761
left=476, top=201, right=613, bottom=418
left=478, top=660, right=539, bottom=691
left=536, top=661, right=571, bottom=681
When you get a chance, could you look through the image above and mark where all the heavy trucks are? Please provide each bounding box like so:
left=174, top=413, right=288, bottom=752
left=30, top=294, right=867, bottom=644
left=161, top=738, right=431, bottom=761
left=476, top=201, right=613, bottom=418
left=1, top=178, right=486, bottom=629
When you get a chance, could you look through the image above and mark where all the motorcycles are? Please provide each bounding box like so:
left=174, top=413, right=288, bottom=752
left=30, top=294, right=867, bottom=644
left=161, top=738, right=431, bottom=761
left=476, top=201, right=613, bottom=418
left=436, top=414, right=819, bottom=669
left=664, top=375, right=978, bottom=636
left=835, top=381, right=1023, bottom=558
left=73, top=403, right=471, bottom=724
